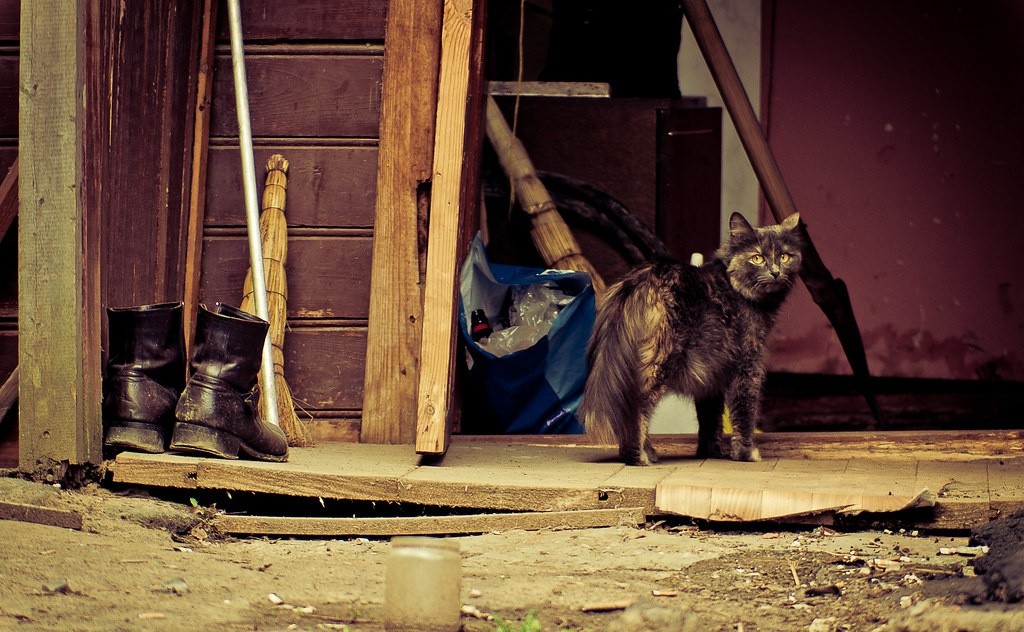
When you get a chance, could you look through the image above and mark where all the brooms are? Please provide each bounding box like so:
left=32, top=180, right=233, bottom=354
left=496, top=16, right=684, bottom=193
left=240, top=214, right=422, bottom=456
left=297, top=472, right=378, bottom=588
left=230, top=154, right=323, bottom=452
left=476, top=90, right=613, bottom=305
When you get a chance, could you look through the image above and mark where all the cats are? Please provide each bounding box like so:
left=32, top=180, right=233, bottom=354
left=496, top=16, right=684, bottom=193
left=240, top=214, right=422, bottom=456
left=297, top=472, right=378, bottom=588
left=578, top=211, right=804, bottom=469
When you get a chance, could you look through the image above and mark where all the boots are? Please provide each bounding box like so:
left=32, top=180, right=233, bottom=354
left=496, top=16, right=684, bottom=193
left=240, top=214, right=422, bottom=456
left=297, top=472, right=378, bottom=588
left=104, top=301, right=187, bottom=453
left=170, top=302, right=289, bottom=463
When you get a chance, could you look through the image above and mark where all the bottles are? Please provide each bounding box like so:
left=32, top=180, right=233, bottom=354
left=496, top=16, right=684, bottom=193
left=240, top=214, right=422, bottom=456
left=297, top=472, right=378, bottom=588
left=472, top=309, right=492, bottom=343
left=384, top=537, right=462, bottom=632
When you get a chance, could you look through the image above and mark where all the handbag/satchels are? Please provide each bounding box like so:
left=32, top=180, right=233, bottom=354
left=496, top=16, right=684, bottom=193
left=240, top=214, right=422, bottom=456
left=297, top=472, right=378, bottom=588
left=455, top=230, right=596, bottom=435
